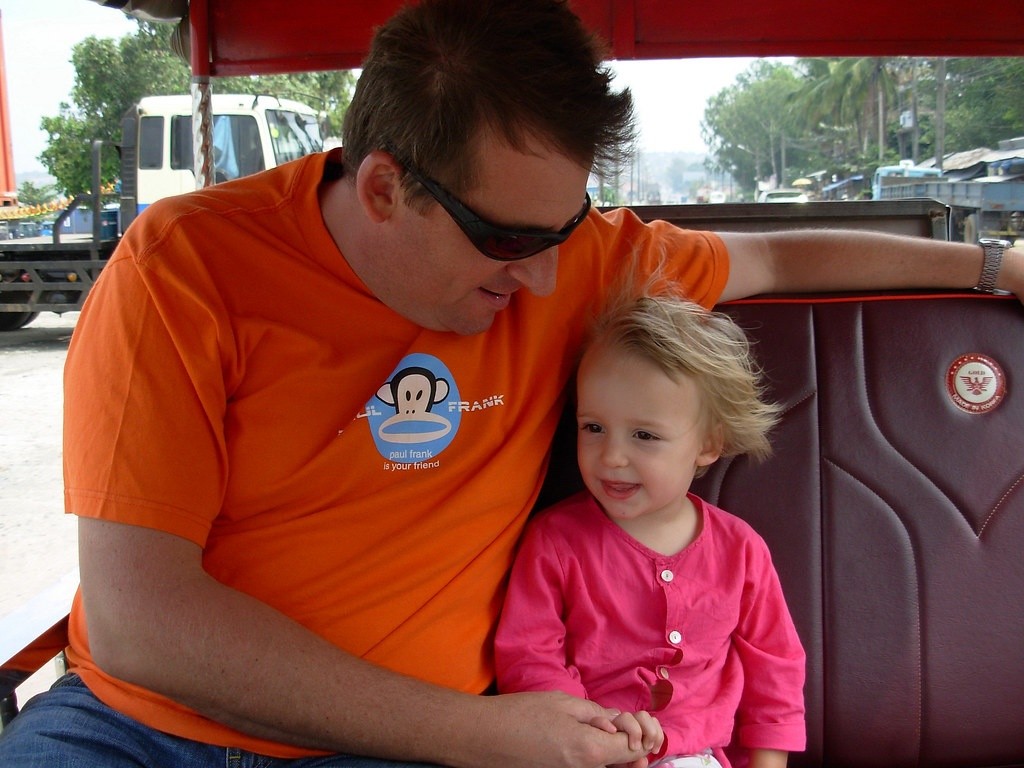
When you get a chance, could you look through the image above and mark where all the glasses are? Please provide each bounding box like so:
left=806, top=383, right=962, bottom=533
left=380, top=134, right=592, bottom=261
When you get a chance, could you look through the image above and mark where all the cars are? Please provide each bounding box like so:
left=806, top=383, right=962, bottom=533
left=757, top=189, right=809, bottom=206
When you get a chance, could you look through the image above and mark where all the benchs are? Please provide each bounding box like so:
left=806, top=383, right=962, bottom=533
left=1, top=282, right=1024, bottom=768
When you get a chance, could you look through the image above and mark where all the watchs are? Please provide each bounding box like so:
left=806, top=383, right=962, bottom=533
left=974, top=237, right=1013, bottom=294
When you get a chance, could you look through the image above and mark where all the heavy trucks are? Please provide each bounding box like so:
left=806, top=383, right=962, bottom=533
left=870, top=166, right=1024, bottom=245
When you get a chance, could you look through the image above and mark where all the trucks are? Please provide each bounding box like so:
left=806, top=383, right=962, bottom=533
left=0, top=92, right=330, bottom=333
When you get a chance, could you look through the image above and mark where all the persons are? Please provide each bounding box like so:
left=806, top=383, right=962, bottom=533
left=495, top=297, right=809, bottom=768
left=1, top=0, right=1023, bottom=768
